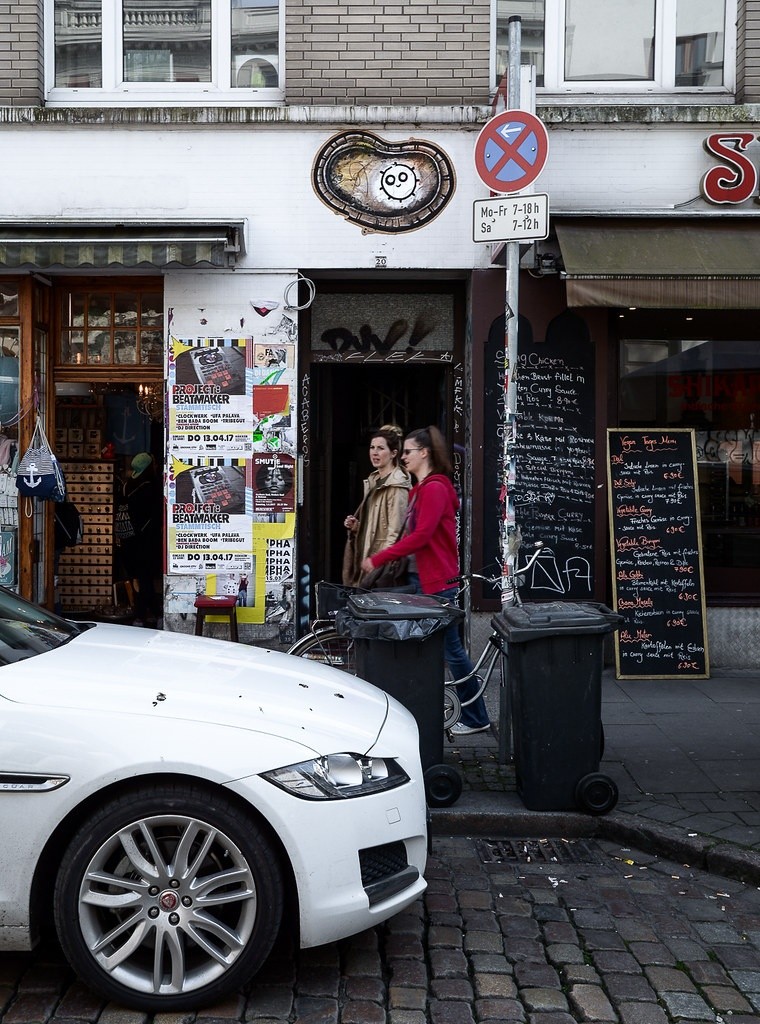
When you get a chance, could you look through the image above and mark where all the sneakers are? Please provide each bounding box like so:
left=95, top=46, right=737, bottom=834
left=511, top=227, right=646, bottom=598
left=450, top=721, right=491, bottom=736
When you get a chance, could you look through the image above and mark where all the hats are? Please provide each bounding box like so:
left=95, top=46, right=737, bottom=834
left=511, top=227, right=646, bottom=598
left=131, top=453, right=152, bottom=479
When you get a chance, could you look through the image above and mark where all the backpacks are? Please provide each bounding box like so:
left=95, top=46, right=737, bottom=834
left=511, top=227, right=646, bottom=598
left=114, top=481, right=150, bottom=539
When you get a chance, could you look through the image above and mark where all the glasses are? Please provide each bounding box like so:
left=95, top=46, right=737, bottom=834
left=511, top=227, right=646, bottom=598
left=403, top=449, right=421, bottom=455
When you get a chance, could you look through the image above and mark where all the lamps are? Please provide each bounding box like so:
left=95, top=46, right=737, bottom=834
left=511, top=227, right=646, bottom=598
left=136, top=382, right=163, bottom=424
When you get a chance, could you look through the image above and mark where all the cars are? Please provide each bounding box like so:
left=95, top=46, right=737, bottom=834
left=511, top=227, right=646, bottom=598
left=0, top=587, right=428, bottom=1012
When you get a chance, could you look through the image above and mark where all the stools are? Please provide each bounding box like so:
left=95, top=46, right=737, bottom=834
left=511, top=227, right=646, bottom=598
left=194, top=595, right=239, bottom=642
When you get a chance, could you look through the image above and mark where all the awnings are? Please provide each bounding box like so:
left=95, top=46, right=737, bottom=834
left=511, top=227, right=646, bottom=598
left=0, top=228, right=232, bottom=268
left=551, top=215, right=760, bottom=311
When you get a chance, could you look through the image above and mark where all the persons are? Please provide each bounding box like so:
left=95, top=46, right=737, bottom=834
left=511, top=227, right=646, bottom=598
left=343, top=427, right=412, bottom=589
left=261, top=466, right=290, bottom=497
left=361, top=426, right=491, bottom=734
left=117, top=453, right=162, bottom=625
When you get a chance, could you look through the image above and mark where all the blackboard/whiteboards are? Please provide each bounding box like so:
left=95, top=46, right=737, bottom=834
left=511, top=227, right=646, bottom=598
left=481, top=339, right=599, bottom=604
left=606, top=426, right=710, bottom=680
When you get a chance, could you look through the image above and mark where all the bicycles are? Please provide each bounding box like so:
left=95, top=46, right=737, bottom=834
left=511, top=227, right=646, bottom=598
left=285, top=538, right=554, bottom=742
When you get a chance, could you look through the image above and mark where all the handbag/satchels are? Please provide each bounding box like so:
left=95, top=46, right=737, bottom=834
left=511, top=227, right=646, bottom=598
left=16, top=416, right=66, bottom=503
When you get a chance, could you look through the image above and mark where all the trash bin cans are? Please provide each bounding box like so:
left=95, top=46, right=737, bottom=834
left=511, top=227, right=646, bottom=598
left=491, top=599, right=625, bottom=815
left=343, top=591, right=464, bottom=809
